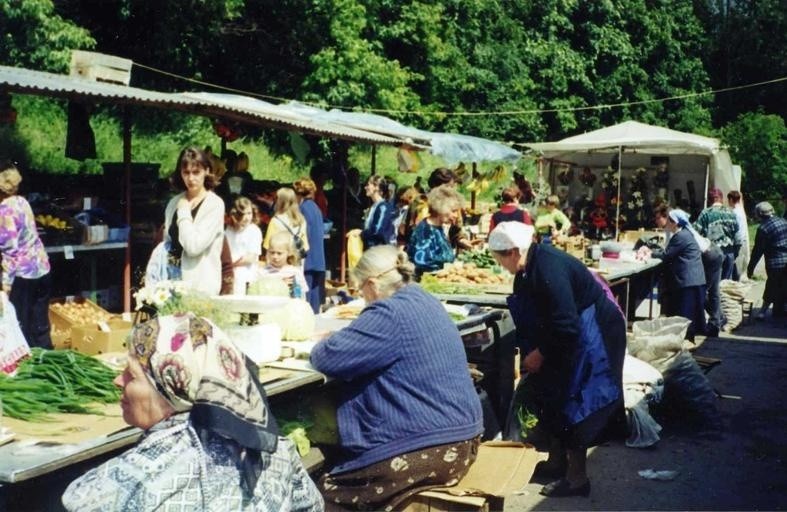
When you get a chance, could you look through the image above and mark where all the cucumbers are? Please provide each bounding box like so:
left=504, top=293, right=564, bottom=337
left=456, top=245, right=501, bottom=270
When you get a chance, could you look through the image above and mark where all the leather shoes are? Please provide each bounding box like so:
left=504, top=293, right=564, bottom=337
left=536, top=460, right=591, bottom=497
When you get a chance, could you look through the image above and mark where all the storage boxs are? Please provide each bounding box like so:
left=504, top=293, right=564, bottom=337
left=69, top=320, right=135, bottom=357
left=47, top=296, right=108, bottom=337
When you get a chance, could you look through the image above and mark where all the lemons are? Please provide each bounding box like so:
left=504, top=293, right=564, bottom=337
left=33, top=213, right=68, bottom=231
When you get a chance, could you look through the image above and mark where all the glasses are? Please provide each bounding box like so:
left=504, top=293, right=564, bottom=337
left=356, top=276, right=378, bottom=297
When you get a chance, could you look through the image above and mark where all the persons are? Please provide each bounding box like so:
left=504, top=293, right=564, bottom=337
left=147, top=145, right=577, bottom=314
left=747, top=201, right=787, bottom=325
left=55, top=311, right=325, bottom=512
left=651, top=188, right=749, bottom=344
left=486, top=220, right=628, bottom=497
left=314, top=244, right=486, bottom=510
left=0, top=165, right=54, bottom=350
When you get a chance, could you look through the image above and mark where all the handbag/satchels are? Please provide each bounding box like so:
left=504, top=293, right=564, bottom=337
left=294, top=235, right=308, bottom=259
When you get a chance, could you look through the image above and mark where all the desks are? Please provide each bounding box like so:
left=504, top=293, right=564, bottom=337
left=268, top=296, right=504, bottom=386
left=433, top=262, right=631, bottom=335
left=22, top=242, right=128, bottom=335
left=602, top=254, right=663, bottom=321
left=0, top=366, right=322, bottom=486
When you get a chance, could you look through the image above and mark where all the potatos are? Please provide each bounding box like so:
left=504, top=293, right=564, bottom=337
left=431, top=262, right=511, bottom=285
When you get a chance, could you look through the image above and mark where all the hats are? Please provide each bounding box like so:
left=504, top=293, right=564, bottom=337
left=755, top=201, right=775, bottom=218
left=708, top=189, right=723, bottom=198
left=0, top=169, right=22, bottom=195
